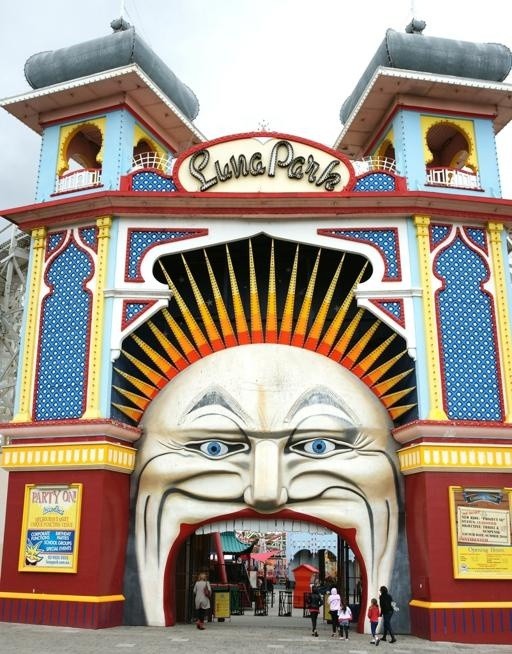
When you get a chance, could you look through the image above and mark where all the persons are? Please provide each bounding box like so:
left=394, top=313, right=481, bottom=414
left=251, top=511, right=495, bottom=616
left=193, top=572, right=212, bottom=630
left=128, top=343, right=398, bottom=634
left=327, top=588, right=341, bottom=635
left=380, top=586, right=396, bottom=643
left=337, top=598, right=353, bottom=640
left=306, top=586, right=323, bottom=634
left=368, top=599, right=380, bottom=646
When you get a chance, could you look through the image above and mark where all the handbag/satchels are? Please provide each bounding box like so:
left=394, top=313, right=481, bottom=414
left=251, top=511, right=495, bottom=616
left=204, top=581, right=211, bottom=598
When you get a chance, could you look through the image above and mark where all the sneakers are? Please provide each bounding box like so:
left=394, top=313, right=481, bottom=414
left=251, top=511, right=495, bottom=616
left=311, top=627, right=350, bottom=642
left=374, top=637, right=380, bottom=646
left=195, top=619, right=200, bottom=629
left=388, top=636, right=397, bottom=643
left=199, top=623, right=206, bottom=630
left=369, top=639, right=375, bottom=644
left=380, top=635, right=387, bottom=641
